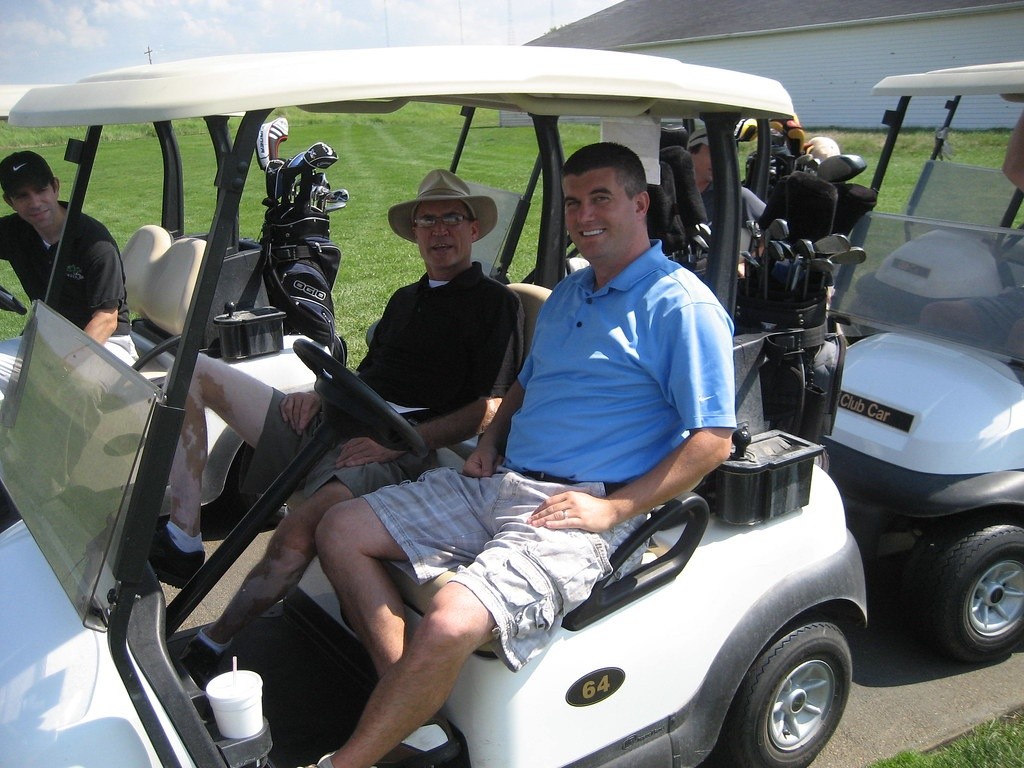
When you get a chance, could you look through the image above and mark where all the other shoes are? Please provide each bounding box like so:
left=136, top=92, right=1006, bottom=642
left=372, top=715, right=458, bottom=768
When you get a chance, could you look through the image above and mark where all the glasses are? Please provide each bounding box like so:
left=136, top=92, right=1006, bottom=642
left=414, top=213, right=476, bottom=227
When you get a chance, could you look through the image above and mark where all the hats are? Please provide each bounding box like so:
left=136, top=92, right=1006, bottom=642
left=388, top=169, right=497, bottom=243
left=688, top=127, right=712, bottom=151
left=0, top=150, right=52, bottom=194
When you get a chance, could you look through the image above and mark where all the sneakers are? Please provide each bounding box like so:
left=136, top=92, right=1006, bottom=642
left=107, top=508, right=205, bottom=589
left=178, top=633, right=224, bottom=676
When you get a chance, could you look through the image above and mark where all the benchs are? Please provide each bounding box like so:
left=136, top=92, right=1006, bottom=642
left=284, top=283, right=559, bottom=658
left=121, top=225, right=207, bottom=386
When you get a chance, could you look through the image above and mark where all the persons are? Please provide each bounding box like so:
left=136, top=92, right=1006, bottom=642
left=315, top=142, right=736, bottom=766
left=1, top=151, right=139, bottom=502
left=147, top=170, right=525, bottom=689
left=685, top=127, right=767, bottom=231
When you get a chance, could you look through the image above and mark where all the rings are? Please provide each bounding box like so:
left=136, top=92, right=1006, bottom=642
left=562, top=510, right=568, bottom=519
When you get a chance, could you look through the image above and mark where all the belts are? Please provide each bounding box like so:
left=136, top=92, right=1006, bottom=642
left=407, top=414, right=421, bottom=426
left=513, top=468, right=621, bottom=498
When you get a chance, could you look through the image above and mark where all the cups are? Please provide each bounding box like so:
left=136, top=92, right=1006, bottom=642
left=206, top=669, right=263, bottom=740
